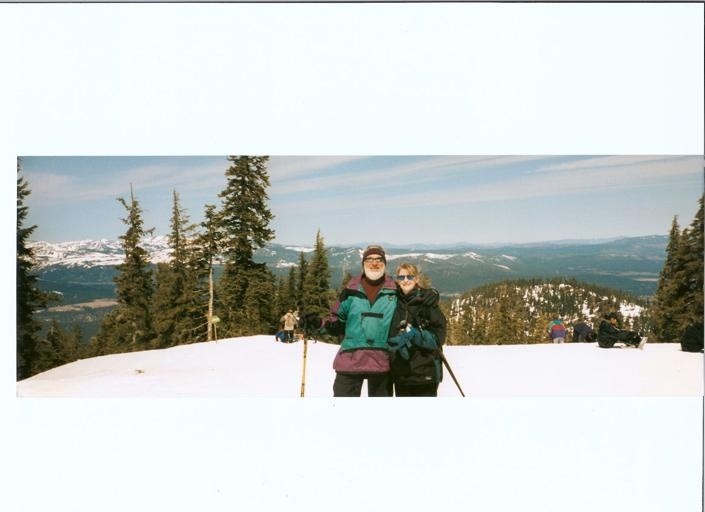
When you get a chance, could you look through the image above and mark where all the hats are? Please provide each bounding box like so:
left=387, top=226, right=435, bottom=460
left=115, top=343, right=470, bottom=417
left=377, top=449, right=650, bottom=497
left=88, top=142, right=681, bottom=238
left=362, top=245, right=386, bottom=266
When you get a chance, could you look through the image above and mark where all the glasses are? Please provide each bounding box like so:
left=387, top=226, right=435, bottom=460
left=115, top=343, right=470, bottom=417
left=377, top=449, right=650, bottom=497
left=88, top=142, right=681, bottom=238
left=364, top=257, right=384, bottom=263
left=398, top=275, right=414, bottom=281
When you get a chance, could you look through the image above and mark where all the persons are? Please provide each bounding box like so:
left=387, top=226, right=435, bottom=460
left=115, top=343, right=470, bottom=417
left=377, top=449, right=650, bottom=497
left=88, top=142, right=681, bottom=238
left=275, top=328, right=300, bottom=342
left=680, top=317, right=704, bottom=352
left=546, top=318, right=566, bottom=344
left=572, top=322, right=597, bottom=342
left=279, top=309, right=299, bottom=342
left=300, top=244, right=440, bottom=396
left=388, top=259, right=447, bottom=396
left=597, top=312, right=649, bottom=348
